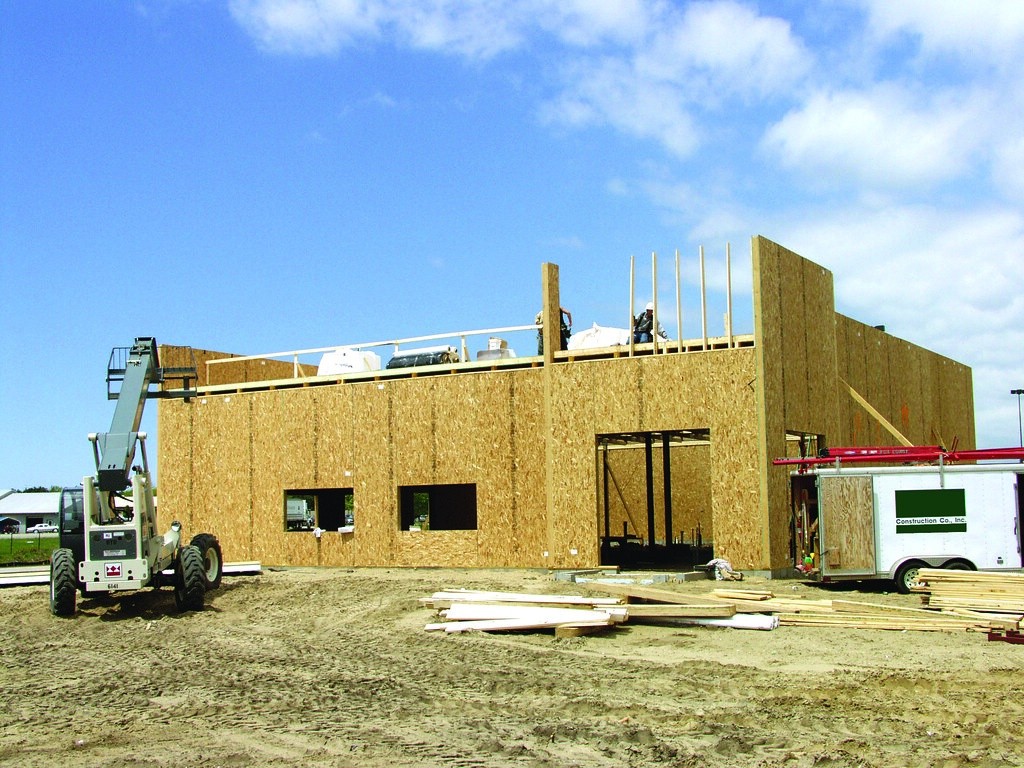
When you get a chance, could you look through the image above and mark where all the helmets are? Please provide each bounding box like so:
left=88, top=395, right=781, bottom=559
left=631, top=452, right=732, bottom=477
left=646, top=303, right=654, bottom=310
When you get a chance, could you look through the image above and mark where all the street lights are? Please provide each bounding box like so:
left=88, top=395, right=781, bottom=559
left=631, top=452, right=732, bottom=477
left=1010, top=389, right=1024, bottom=448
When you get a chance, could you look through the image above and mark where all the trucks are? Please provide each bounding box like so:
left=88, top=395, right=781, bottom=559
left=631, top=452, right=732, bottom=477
left=786, top=454, right=1024, bottom=595
left=286, top=497, right=312, bottom=531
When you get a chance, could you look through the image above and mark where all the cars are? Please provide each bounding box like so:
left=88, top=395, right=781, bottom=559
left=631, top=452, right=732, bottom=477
left=345, top=514, right=353, bottom=525
left=419, top=514, right=426, bottom=522
left=26, top=523, right=60, bottom=533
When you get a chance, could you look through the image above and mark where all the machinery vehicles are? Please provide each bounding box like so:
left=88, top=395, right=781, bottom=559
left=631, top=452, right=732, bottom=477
left=49, top=336, right=224, bottom=617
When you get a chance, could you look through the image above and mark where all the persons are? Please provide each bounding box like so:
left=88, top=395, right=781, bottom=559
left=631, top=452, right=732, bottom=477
left=3, top=524, right=11, bottom=533
left=535, top=304, right=572, bottom=355
left=626, top=302, right=670, bottom=345
